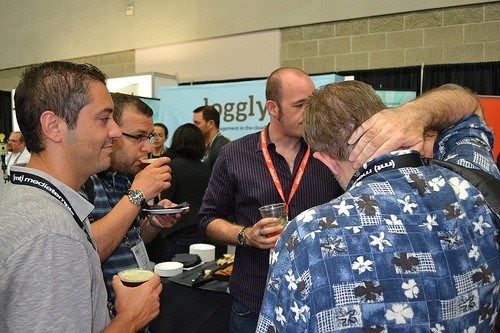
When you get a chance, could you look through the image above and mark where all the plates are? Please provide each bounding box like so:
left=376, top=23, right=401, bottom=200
left=143, top=207, right=189, bottom=214
left=154, top=262, right=184, bottom=278
left=189, top=243, right=215, bottom=262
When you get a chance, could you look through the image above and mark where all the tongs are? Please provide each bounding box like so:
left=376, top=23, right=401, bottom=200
left=192, top=269, right=225, bottom=288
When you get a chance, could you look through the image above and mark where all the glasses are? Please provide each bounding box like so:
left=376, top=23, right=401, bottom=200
left=121, top=132, right=154, bottom=143
left=6, top=139, right=21, bottom=143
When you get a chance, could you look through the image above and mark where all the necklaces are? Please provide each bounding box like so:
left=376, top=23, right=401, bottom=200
left=6, top=149, right=25, bottom=167
left=7, top=170, right=114, bottom=319
left=203, top=131, right=221, bottom=163
left=261, top=126, right=310, bottom=223
left=345, top=152, right=424, bottom=188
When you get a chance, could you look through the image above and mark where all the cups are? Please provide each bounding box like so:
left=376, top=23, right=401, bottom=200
left=115, top=261, right=156, bottom=288
left=140, top=157, right=159, bottom=170
left=258, top=203, right=286, bottom=238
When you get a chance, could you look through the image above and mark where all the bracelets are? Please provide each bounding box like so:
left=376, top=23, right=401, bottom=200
left=148, top=214, right=157, bottom=228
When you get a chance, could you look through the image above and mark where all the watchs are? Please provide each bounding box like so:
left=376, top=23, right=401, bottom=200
left=237, top=227, right=249, bottom=248
left=125, top=188, right=145, bottom=207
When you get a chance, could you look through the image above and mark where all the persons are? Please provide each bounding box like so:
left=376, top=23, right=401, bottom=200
left=193, top=106, right=230, bottom=174
left=257, top=80, right=500, bottom=333
left=0, top=61, right=163, bottom=333
left=77, top=92, right=190, bottom=333
left=148, top=123, right=169, bottom=159
left=158, top=123, right=212, bottom=264
left=197, top=66, right=345, bottom=333
left=0, top=131, right=30, bottom=176
